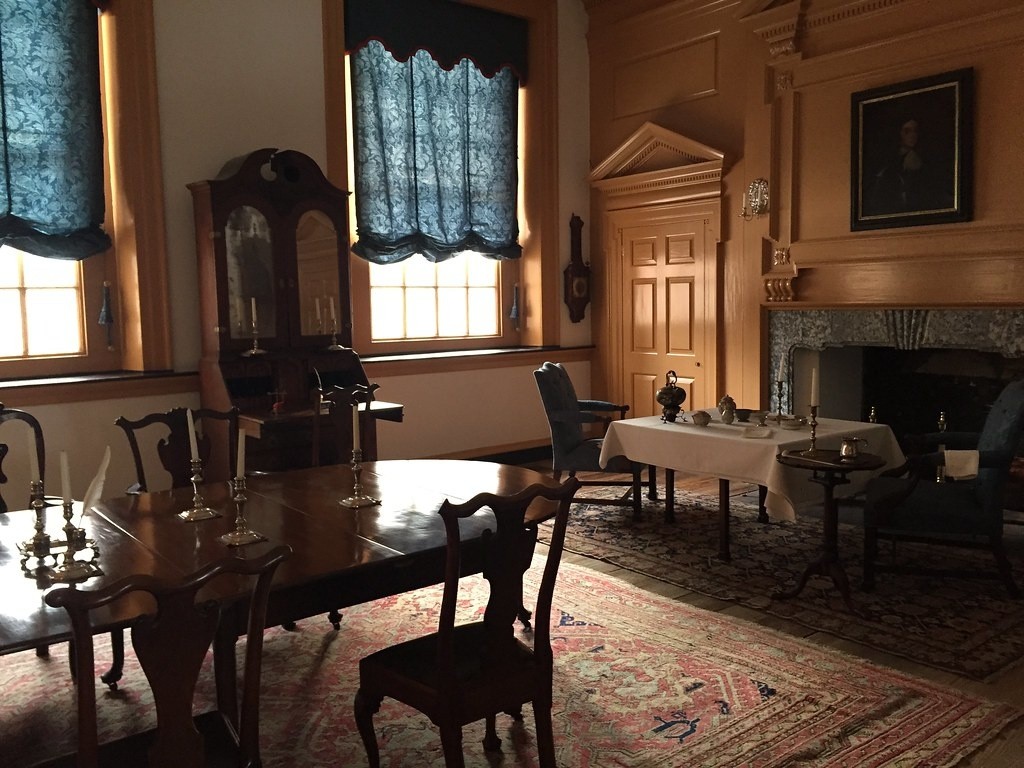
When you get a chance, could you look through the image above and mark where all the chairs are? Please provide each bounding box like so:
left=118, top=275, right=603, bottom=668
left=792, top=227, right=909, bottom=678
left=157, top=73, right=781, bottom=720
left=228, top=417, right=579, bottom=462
left=862, top=370, right=1011, bottom=477
left=859, top=381, right=1024, bottom=598
left=532, top=361, right=657, bottom=521
left=0, top=401, right=583, bottom=768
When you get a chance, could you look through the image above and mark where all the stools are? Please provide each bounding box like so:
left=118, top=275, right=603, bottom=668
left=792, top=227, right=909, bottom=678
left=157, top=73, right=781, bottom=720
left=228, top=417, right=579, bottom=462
left=1003, top=456, right=1024, bottom=510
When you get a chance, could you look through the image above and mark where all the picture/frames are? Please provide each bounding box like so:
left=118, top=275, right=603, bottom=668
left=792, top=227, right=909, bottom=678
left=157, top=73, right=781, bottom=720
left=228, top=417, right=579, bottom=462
left=850, top=67, right=974, bottom=231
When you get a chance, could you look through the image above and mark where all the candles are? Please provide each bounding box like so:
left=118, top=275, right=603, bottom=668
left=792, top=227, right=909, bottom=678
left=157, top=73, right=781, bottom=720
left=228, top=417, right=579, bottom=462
left=329, top=296, right=335, bottom=320
left=27, top=427, right=40, bottom=484
left=353, top=399, right=360, bottom=451
left=779, top=353, right=784, bottom=380
left=60, top=450, right=72, bottom=503
left=237, top=429, right=245, bottom=479
left=315, top=297, right=320, bottom=320
left=251, top=297, right=257, bottom=328
left=810, top=367, right=820, bottom=407
left=758, top=184, right=761, bottom=206
left=743, top=193, right=745, bottom=208
left=186, top=408, right=199, bottom=460
left=236, top=296, right=241, bottom=321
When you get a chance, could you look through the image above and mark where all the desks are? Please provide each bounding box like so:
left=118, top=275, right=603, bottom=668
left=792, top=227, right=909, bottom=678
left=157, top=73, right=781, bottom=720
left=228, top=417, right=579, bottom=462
left=613, top=408, right=890, bottom=596
left=0, top=501, right=222, bottom=768
left=91, top=460, right=562, bottom=768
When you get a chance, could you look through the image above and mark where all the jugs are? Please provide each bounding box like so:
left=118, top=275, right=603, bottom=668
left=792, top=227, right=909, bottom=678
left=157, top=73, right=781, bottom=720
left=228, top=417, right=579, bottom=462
left=838, top=436, right=868, bottom=464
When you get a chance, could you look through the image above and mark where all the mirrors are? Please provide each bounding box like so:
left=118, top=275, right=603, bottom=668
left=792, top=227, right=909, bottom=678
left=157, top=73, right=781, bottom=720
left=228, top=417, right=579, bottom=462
left=223, top=204, right=276, bottom=339
left=295, top=208, right=342, bottom=336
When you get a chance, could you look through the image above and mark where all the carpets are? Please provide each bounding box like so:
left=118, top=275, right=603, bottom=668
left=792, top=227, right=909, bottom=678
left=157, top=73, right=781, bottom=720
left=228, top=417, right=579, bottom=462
left=0, top=545, right=1024, bottom=768
left=525, top=480, right=1024, bottom=685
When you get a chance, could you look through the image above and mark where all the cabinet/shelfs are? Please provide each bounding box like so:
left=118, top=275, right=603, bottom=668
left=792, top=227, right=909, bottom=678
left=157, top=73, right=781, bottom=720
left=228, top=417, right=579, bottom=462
left=213, top=196, right=349, bottom=347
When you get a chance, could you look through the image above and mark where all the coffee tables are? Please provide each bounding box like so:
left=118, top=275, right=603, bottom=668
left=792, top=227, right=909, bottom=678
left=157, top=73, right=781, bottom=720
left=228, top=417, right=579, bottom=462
left=762, top=449, right=901, bottom=621
